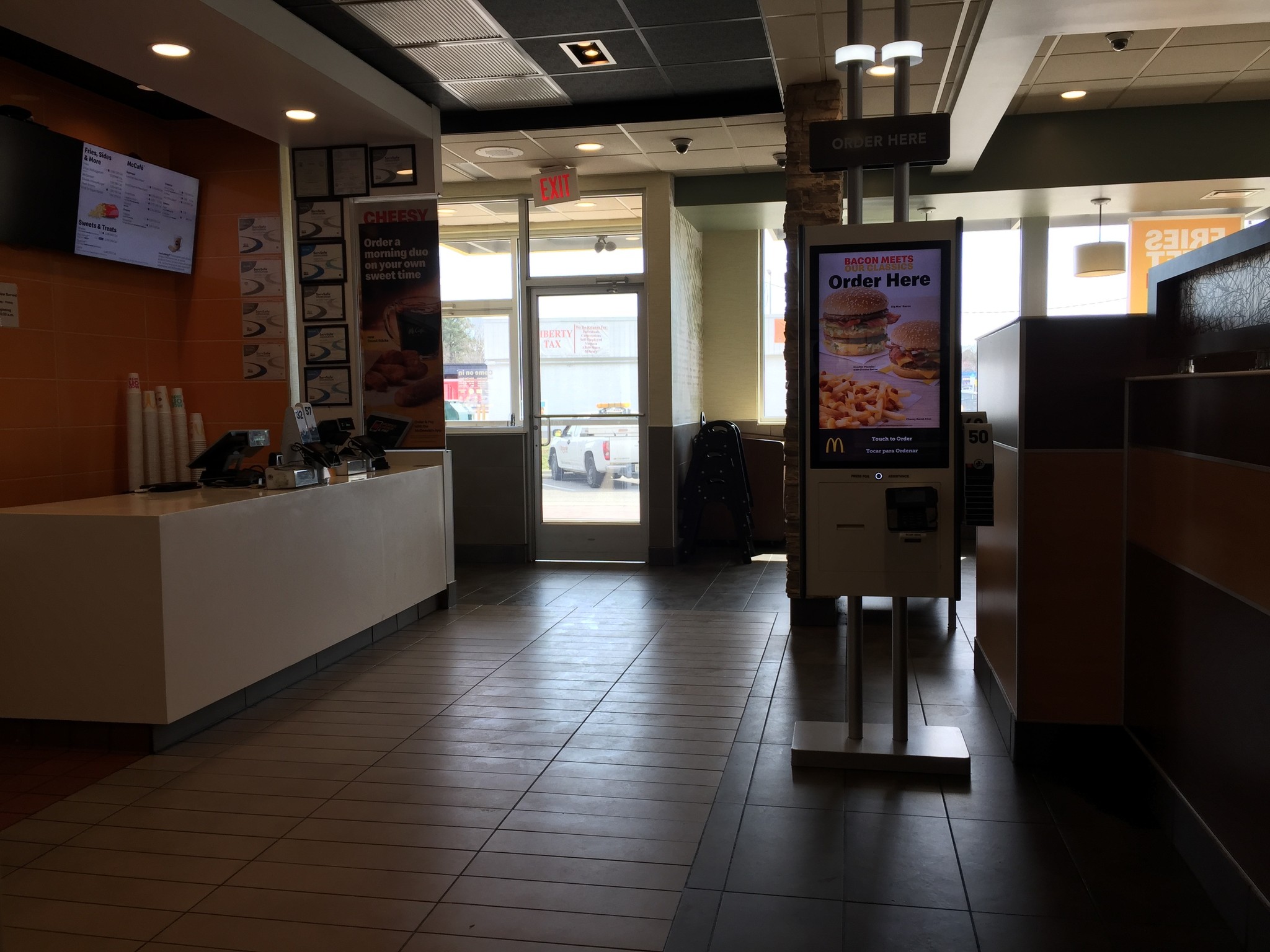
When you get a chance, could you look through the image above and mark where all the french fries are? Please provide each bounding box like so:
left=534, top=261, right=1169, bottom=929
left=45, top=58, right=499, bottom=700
left=818, top=370, right=912, bottom=429
left=87, top=203, right=106, bottom=218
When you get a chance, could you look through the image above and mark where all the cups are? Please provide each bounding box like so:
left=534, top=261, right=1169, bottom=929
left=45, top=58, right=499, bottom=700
left=188, top=413, right=207, bottom=486
left=127, top=373, right=144, bottom=492
left=142, top=391, right=161, bottom=485
left=155, top=386, right=176, bottom=483
left=171, top=388, right=191, bottom=482
left=383, top=296, right=441, bottom=361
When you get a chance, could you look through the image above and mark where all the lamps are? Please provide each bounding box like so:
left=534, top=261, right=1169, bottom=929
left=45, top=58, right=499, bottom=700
left=594, top=236, right=617, bottom=253
left=1073, top=198, right=1125, bottom=276
left=531, top=165, right=581, bottom=207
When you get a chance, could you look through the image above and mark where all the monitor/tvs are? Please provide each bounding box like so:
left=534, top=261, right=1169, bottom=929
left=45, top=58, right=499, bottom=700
left=186, top=429, right=270, bottom=487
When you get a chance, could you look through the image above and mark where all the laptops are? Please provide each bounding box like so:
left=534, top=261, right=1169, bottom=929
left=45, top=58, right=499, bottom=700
left=139, top=479, right=197, bottom=494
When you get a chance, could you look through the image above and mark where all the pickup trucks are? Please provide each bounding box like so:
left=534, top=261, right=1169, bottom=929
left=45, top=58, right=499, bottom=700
left=547, top=424, right=639, bottom=488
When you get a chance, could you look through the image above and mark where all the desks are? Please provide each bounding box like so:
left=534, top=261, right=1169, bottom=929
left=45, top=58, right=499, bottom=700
left=0, top=462, right=444, bottom=758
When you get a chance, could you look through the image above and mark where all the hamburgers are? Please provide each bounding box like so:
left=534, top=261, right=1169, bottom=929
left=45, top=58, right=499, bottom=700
left=818, top=286, right=903, bottom=356
left=880, top=320, right=941, bottom=380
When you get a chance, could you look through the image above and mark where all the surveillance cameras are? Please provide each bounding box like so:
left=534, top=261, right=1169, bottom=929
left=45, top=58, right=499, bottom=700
left=1105, top=31, right=1134, bottom=53
left=772, top=152, right=788, bottom=169
left=671, top=137, right=693, bottom=155
